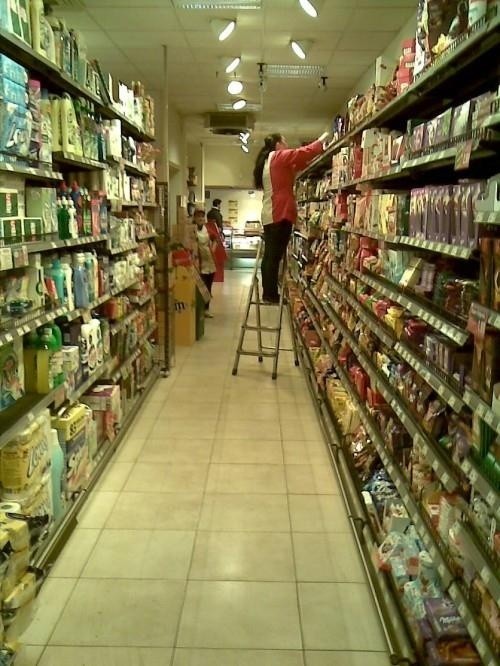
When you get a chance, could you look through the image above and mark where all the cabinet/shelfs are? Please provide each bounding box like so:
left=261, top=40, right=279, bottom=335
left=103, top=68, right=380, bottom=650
left=278, top=10, right=500, bottom=666
left=0, top=23, right=171, bottom=625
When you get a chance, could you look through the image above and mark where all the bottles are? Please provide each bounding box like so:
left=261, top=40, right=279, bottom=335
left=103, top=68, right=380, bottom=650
left=58, top=179, right=93, bottom=239
left=44, top=250, right=99, bottom=311
left=51, top=428, right=65, bottom=518
left=28, top=320, right=62, bottom=393
left=86, top=409, right=97, bottom=460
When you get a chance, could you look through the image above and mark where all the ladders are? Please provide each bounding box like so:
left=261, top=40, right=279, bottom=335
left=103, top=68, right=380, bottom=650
left=231, top=230, right=299, bottom=379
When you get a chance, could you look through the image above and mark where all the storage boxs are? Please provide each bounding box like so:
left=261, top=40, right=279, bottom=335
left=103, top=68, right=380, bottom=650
left=169, top=202, right=213, bottom=349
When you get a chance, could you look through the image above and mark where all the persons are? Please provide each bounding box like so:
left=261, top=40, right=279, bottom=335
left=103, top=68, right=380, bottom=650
left=190, top=207, right=218, bottom=318
left=252, top=130, right=331, bottom=304
left=206, top=199, right=223, bottom=234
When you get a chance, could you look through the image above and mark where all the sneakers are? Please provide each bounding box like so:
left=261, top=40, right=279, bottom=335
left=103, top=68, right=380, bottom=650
left=263, top=293, right=287, bottom=304
left=204, top=310, right=213, bottom=317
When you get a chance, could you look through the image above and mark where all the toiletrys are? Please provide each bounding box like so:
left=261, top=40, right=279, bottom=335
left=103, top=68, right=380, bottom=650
left=0, top=0, right=123, bottom=240
left=62, top=317, right=112, bottom=390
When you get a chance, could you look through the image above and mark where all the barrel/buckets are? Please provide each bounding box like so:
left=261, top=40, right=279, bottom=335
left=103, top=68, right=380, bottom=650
left=42, top=249, right=106, bottom=310
left=49, top=427, right=69, bottom=524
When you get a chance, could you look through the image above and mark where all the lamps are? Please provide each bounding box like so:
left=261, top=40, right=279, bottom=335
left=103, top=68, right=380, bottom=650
left=208, top=17, right=251, bottom=154
left=299, top=0, right=321, bottom=18
